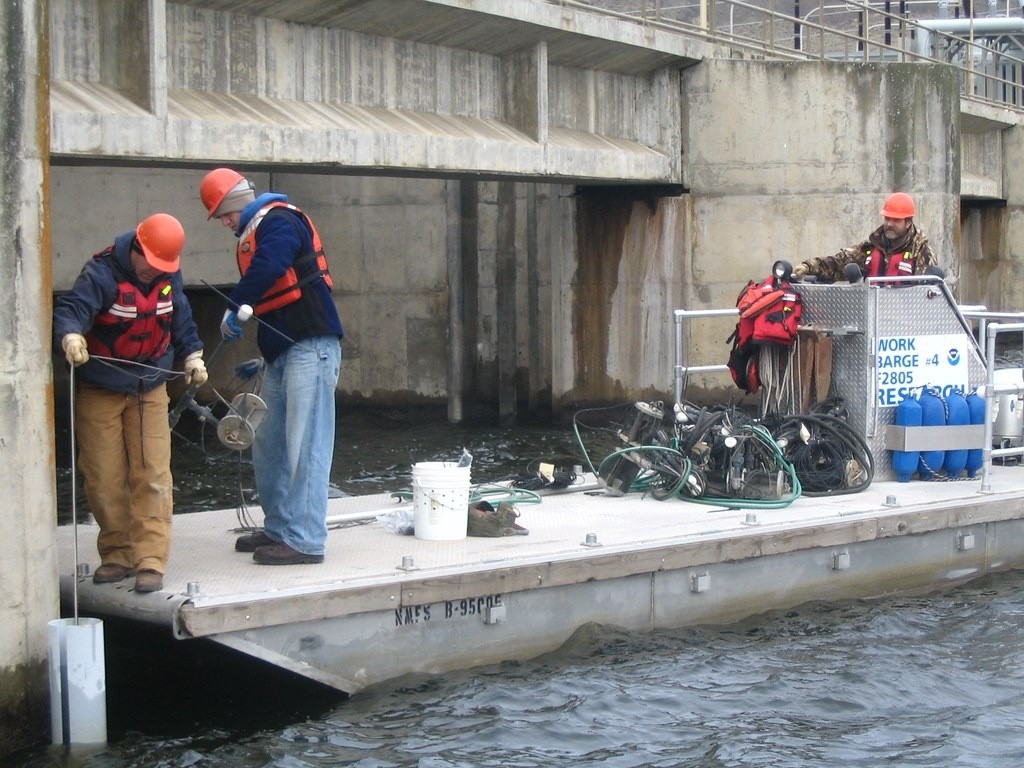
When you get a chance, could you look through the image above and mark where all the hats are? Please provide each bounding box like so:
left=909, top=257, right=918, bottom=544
left=211, top=177, right=254, bottom=218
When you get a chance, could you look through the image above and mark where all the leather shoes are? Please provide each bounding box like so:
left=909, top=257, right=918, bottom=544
left=236, top=531, right=278, bottom=551
left=253, top=541, right=324, bottom=565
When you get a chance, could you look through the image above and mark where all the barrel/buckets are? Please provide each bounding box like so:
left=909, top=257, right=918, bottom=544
left=409, top=461, right=472, bottom=541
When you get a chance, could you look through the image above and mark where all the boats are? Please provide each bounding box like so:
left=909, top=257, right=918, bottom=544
left=43, top=271, right=1024, bottom=701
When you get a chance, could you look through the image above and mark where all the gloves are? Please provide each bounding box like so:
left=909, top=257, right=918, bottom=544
left=793, top=265, right=807, bottom=276
left=184, top=349, right=208, bottom=388
left=61, top=333, right=89, bottom=367
left=220, top=309, right=243, bottom=341
left=234, top=359, right=261, bottom=380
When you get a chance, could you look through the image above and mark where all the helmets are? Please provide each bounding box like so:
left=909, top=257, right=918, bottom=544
left=137, top=214, right=185, bottom=274
left=200, top=168, right=245, bottom=221
left=881, top=192, right=914, bottom=219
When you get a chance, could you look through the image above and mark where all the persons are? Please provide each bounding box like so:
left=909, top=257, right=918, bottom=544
left=791, top=192, right=938, bottom=286
left=52, top=213, right=209, bottom=591
left=200, top=168, right=344, bottom=566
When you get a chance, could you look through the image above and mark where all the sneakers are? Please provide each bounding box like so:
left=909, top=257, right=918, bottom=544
left=135, top=568, right=163, bottom=591
left=92, top=562, right=134, bottom=582
